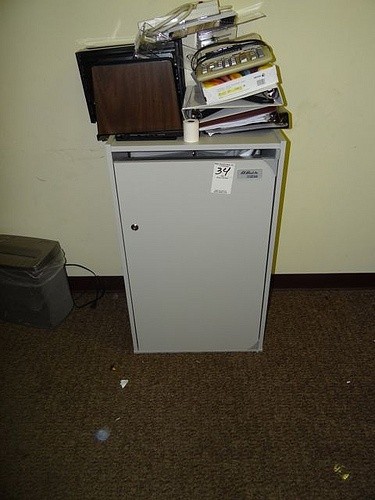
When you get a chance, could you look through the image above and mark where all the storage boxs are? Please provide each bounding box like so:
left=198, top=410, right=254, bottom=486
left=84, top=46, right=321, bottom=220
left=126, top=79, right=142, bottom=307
left=199, top=64, right=280, bottom=105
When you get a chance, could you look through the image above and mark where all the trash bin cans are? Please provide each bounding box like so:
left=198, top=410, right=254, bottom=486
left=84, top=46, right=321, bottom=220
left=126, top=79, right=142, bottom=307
left=1, top=233, right=74, bottom=330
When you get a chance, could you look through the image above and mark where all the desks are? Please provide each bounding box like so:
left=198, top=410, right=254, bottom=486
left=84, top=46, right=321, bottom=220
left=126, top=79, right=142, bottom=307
left=105, top=124, right=287, bottom=358
